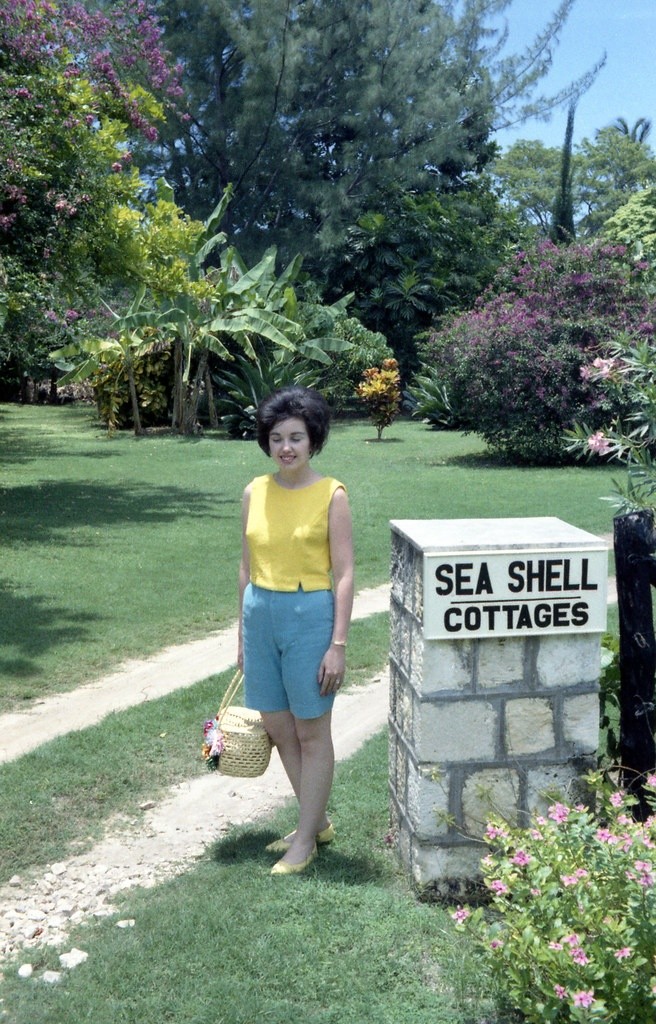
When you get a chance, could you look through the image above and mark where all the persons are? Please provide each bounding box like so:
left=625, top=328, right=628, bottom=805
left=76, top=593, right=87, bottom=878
left=238, top=385, right=354, bottom=876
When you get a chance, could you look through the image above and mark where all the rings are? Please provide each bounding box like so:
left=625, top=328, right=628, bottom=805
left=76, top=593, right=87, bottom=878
left=336, top=680, right=339, bottom=684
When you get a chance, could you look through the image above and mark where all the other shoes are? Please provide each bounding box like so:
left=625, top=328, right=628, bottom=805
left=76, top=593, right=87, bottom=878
left=271, top=841, right=317, bottom=876
left=266, top=823, right=335, bottom=853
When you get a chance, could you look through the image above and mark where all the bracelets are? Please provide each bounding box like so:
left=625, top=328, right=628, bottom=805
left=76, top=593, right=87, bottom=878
left=331, top=641, right=347, bottom=646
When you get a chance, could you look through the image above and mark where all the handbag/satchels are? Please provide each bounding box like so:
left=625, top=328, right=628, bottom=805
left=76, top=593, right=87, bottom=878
left=201, top=669, right=272, bottom=778
